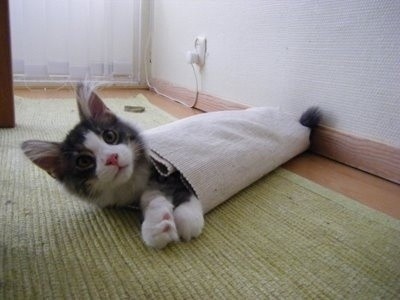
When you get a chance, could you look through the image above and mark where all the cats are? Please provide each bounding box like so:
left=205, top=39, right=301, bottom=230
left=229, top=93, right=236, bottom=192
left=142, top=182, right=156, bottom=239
left=19, top=70, right=325, bottom=251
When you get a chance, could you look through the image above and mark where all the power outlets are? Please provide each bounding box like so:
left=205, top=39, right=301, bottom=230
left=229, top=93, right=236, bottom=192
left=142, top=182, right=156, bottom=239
left=193, top=37, right=206, bottom=66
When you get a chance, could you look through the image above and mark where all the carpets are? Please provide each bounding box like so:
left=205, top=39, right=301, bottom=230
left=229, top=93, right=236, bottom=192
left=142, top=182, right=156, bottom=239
left=1, top=92, right=400, bottom=300
